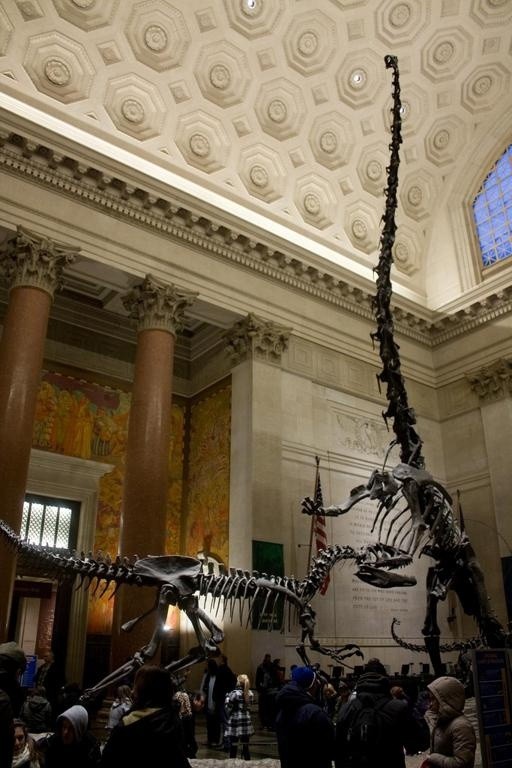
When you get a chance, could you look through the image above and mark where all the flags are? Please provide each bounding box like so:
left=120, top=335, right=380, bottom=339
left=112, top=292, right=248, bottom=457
left=313, top=472, right=331, bottom=596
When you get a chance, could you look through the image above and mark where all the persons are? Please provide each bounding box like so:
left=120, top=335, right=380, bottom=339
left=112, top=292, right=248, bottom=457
left=102, top=665, right=191, bottom=768
left=201, top=656, right=255, bottom=760
left=274, top=666, right=336, bottom=768
left=425, top=676, right=477, bottom=768
left=336, top=658, right=424, bottom=768
left=105, top=685, right=198, bottom=760
left=257, top=654, right=432, bottom=756
left=1, top=640, right=102, bottom=768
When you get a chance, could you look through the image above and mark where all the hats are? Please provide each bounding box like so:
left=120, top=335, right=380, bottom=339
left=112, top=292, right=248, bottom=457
left=0, top=642, right=28, bottom=674
left=292, top=666, right=316, bottom=690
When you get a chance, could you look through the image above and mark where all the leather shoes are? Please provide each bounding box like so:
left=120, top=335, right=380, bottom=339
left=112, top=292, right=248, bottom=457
left=201, top=743, right=225, bottom=751
